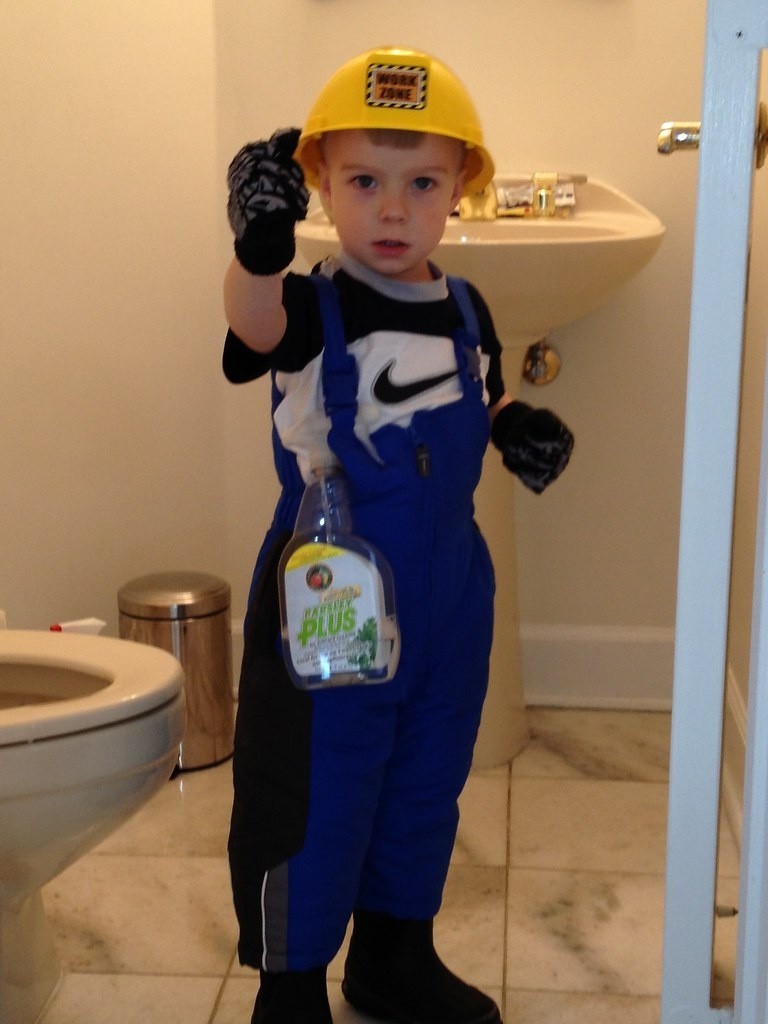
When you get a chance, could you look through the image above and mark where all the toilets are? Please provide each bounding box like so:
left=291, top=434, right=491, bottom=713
left=1, top=629, right=187, bottom=1024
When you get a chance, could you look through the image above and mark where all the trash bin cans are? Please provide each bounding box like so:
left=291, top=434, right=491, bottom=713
left=117, top=571, right=235, bottom=781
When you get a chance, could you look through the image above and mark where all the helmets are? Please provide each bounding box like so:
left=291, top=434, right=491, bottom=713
left=294, top=46, right=495, bottom=196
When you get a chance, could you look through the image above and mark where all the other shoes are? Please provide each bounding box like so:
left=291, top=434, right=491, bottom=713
left=250, top=972, right=333, bottom=1024
left=341, top=920, right=503, bottom=1024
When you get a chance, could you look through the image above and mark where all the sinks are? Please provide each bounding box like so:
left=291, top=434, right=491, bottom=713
left=294, top=173, right=666, bottom=353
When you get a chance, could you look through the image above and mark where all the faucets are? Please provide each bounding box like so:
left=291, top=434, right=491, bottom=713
left=459, top=180, right=498, bottom=220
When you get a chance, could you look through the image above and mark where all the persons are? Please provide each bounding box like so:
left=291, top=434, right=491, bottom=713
left=220, top=49, right=576, bottom=1024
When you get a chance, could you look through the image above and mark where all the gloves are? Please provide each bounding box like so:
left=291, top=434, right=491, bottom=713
left=227, top=127, right=310, bottom=277
left=491, top=399, right=575, bottom=495
